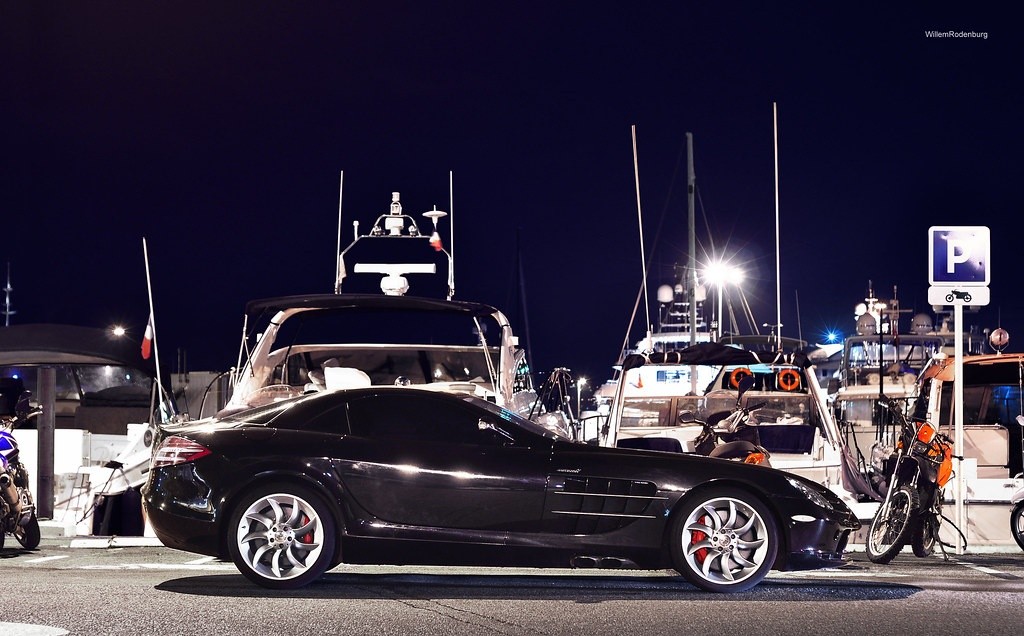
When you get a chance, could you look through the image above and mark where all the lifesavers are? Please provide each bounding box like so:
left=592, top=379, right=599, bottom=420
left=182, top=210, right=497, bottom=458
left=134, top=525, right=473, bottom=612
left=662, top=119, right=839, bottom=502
left=778, top=368, right=801, bottom=392
left=729, top=367, right=751, bottom=389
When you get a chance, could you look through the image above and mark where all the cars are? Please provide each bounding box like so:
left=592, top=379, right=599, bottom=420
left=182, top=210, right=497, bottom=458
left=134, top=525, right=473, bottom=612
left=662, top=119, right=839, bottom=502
left=140, top=387, right=861, bottom=594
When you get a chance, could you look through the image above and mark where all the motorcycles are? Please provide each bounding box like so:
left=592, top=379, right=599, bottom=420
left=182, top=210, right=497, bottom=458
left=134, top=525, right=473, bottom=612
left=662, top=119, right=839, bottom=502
left=1009, top=415, right=1024, bottom=552
left=865, top=394, right=965, bottom=565
left=0, top=390, right=43, bottom=555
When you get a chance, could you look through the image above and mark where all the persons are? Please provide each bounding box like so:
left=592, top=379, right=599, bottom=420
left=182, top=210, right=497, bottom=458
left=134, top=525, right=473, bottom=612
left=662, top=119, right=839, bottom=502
left=0, top=416, right=19, bottom=470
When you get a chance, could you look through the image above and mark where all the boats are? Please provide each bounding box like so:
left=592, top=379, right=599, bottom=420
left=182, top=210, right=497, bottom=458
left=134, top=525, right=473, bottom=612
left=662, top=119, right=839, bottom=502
left=0, top=100, right=1023, bottom=503
